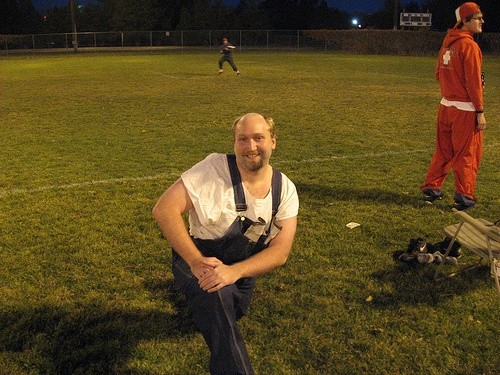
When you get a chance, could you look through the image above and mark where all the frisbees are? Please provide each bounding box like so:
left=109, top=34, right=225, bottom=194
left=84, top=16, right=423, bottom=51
left=227, top=46, right=235, bottom=48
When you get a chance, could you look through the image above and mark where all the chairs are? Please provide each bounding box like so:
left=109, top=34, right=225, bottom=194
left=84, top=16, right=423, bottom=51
left=431, top=206, right=500, bottom=291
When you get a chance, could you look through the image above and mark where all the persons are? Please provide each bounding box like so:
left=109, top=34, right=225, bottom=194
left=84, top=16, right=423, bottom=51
left=153, top=113, right=299, bottom=375
left=422, top=2, right=486, bottom=210
left=218, top=38, right=240, bottom=76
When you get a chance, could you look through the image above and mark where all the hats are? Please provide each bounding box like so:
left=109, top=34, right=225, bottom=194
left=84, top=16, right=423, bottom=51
left=223, top=38, right=227, bottom=40
left=453, top=2, right=481, bottom=30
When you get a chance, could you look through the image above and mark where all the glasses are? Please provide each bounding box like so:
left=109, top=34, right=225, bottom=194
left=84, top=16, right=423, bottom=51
left=468, top=17, right=482, bottom=21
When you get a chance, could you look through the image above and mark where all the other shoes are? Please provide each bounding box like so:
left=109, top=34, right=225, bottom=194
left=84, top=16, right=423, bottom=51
left=417, top=253, right=458, bottom=267
left=236, top=71, right=240, bottom=76
left=170, top=285, right=186, bottom=304
left=219, top=70, right=223, bottom=74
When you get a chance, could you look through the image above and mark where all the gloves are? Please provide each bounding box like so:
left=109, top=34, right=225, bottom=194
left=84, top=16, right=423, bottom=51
left=264, top=216, right=283, bottom=245
left=244, top=217, right=266, bottom=244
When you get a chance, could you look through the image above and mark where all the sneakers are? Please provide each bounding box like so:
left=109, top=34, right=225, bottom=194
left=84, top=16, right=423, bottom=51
left=423, top=192, right=446, bottom=205
left=426, top=235, right=460, bottom=257
left=454, top=197, right=477, bottom=211
left=398, top=237, right=428, bottom=261
